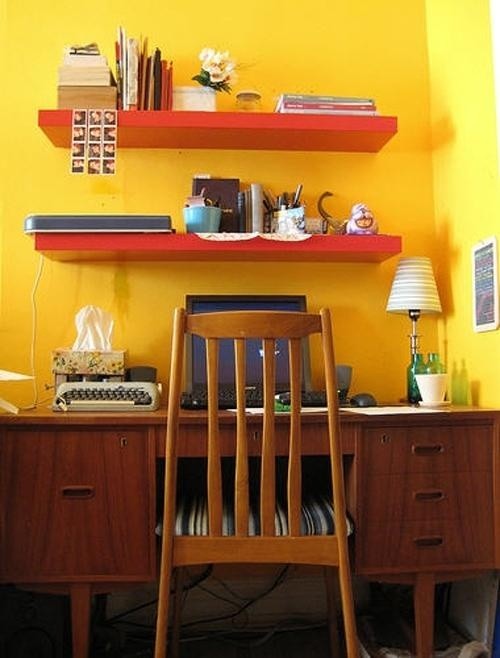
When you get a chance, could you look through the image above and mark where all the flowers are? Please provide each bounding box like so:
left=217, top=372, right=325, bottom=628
left=193, top=48, right=237, bottom=95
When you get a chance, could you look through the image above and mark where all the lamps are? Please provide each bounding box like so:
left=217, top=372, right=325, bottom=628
left=386, top=257, right=443, bottom=355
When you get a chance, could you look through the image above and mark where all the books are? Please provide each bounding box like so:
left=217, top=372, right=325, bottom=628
left=56, top=27, right=173, bottom=111
left=192, top=177, right=264, bottom=233
left=275, top=95, right=378, bottom=116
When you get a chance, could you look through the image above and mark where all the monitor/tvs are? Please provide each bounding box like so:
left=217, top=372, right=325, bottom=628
left=185, top=295, right=313, bottom=391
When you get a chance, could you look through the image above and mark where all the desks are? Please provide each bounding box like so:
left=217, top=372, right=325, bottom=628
left=0, top=404, right=500, bottom=658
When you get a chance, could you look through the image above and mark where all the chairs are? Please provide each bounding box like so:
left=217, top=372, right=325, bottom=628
left=153, top=307, right=360, bottom=658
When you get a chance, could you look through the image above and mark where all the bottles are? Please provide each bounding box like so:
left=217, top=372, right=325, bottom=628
left=450, top=358, right=474, bottom=406
left=406, top=352, right=428, bottom=403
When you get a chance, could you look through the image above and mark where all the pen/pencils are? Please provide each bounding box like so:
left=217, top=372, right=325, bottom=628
left=263, top=184, right=305, bottom=214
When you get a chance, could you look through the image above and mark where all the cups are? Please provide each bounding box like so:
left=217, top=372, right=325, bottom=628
left=414, top=373, right=449, bottom=402
left=184, top=205, right=222, bottom=233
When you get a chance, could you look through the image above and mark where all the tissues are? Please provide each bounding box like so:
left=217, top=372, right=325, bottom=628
left=52, top=305, right=126, bottom=378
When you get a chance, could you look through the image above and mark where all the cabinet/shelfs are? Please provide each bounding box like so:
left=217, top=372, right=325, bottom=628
left=34, top=108, right=402, bottom=262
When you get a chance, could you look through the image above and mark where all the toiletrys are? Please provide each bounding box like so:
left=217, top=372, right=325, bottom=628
left=335, top=365, right=352, bottom=403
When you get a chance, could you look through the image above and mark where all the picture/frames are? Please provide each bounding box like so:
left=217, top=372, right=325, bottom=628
left=473, top=238, right=499, bottom=332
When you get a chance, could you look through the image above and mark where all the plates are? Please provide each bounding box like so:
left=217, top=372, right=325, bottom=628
left=418, top=400, right=451, bottom=407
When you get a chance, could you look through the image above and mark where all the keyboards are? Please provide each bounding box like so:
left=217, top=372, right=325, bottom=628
left=180, top=391, right=350, bottom=410
left=350, top=393, right=377, bottom=408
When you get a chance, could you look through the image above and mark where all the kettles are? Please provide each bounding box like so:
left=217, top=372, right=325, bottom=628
left=424, top=353, right=444, bottom=375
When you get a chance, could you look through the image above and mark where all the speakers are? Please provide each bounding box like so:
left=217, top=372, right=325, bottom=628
left=333, top=365, right=351, bottom=396
